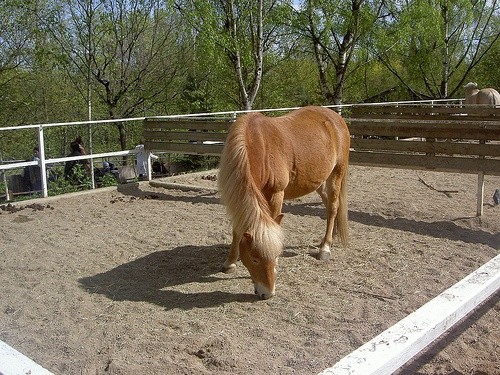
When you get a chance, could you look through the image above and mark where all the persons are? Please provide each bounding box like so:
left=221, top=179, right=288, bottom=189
left=98, top=139, right=158, bottom=181
left=29, top=136, right=90, bottom=179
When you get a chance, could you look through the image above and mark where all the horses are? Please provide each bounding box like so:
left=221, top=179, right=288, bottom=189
left=218, top=105, right=350, bottom=300
left=461, top=82, right=500, bottom=116
